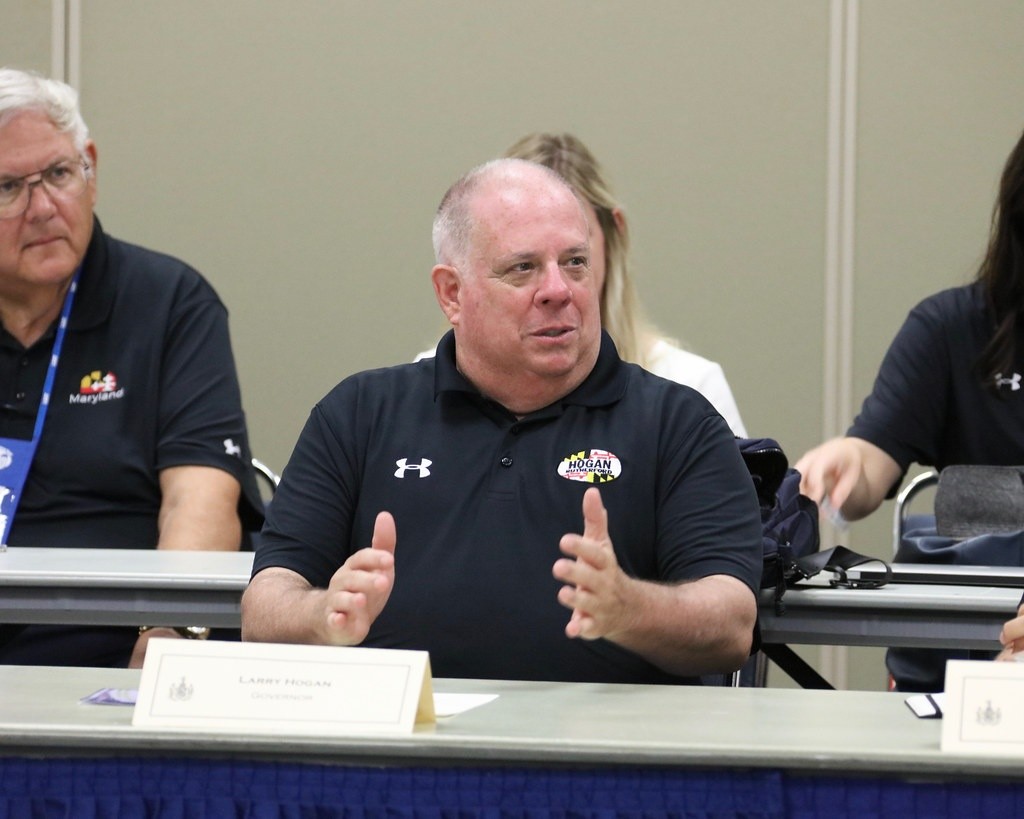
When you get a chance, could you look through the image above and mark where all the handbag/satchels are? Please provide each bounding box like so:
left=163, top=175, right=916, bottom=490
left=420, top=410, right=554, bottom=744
left=885, top=462, right=1024, bottom=691
left=728, top=436, right=891, bottom=618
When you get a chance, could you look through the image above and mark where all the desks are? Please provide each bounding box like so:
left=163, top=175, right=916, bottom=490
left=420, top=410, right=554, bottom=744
left=0, top=547, right=1024, bottom=688
left=0, top=661, right=1024, bottom=819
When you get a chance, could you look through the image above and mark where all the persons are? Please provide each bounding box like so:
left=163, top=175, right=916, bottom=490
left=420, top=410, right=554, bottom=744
left=793, top=133, right=1024, bottom=693
left=0, top=70, right=266, bottom=668
left=242, top=132, right=763, bottom=685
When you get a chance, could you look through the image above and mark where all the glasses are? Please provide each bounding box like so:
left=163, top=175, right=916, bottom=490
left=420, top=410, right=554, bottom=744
left=0, top=153, right=90, bottom=220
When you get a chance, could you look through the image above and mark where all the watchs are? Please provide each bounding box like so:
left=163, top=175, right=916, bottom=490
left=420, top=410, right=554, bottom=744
left=140, top=625, right=210, bottom=640
